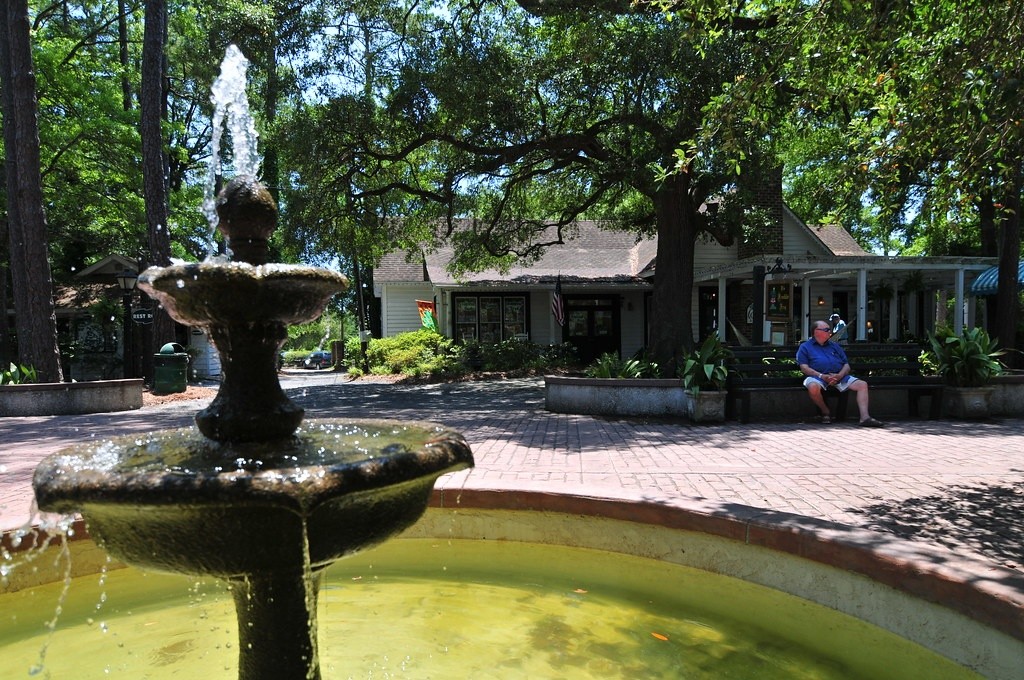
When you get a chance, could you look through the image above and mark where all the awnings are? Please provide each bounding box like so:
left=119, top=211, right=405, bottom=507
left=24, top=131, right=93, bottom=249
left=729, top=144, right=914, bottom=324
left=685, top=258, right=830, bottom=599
left=972, top=261, right=1024, bottom=295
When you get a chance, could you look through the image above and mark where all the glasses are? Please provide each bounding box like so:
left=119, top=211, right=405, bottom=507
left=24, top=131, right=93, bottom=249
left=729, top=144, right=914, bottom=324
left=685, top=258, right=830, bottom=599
left=816, top=328, right=830, bottom=332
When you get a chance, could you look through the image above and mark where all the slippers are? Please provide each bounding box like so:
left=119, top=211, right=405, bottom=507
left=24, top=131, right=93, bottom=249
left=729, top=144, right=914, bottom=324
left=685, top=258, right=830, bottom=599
left=822, top=413, right=831, bottom=424
left=859, top=417, right=883, bottom=427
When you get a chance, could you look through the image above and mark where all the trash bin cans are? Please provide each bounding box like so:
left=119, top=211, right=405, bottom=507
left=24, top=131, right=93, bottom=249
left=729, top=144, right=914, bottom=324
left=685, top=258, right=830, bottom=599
left=152, top=341, right=189, bottom=397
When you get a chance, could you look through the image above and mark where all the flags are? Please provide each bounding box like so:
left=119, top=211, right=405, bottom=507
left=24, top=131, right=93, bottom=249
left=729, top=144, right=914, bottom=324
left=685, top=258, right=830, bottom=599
left=415, top=299, right=439, bottom=333
left=552, top=270, right=565, bottom=327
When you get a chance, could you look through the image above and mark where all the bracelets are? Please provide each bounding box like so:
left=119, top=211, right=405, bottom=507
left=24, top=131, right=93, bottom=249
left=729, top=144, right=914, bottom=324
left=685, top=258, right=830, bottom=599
left=819, top=373, right=822, bottom=378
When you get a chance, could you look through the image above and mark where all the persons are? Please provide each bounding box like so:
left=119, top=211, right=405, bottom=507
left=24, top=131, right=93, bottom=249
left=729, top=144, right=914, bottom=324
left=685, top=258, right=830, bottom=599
left=829, top=314, right=848, bottom=344
left=796, top=321, right=883, bottom=426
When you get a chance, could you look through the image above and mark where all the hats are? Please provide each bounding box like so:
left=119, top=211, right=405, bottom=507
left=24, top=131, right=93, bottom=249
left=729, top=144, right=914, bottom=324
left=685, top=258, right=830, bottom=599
left=829, top=314, right=839, bottom=321
